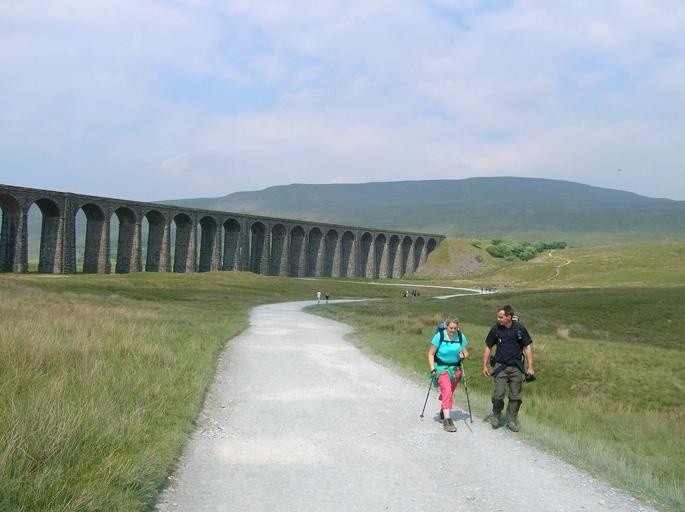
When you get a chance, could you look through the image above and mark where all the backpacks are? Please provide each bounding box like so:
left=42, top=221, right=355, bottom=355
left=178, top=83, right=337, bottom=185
left=434, top=321, right=463, bottom=366
left=490, top=316, right=525, bottom=367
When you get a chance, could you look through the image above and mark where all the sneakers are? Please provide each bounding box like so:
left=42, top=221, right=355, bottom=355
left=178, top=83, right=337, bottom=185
left=440, top=409, right=457, bottom=432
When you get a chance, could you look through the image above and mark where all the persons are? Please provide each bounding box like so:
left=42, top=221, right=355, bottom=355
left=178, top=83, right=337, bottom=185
left=427, top=315, right=469, bottom=432
left=324, top=290, right=329, bottom=304
left=317, top=290, right=321, bottom=304
left=481, top=304, right=535, bottom=432
left=403, top=289, right=421, bottom=298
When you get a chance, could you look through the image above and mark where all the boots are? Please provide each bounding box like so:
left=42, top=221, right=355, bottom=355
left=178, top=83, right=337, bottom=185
left=491, top=397, right=504, bottom=429
left=506, top=399, right=522, bottom=432
